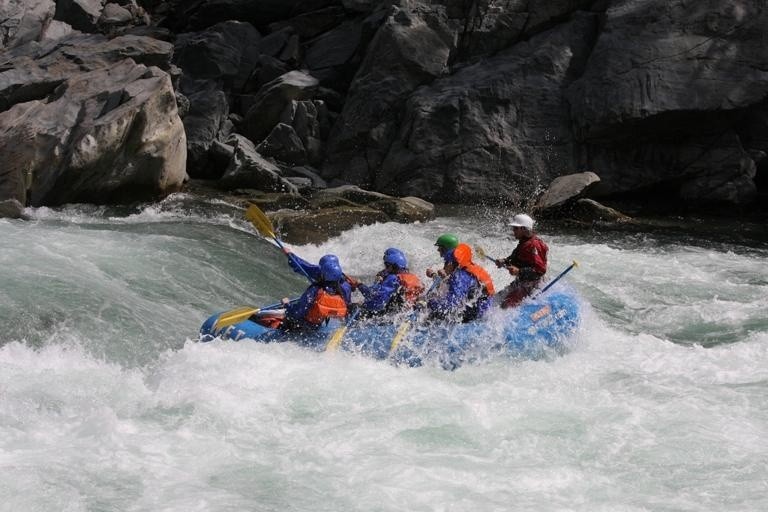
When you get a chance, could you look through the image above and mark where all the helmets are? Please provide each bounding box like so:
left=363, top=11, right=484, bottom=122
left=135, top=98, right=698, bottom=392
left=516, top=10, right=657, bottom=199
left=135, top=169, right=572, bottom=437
left=320, top=261, right=343, bottom=283
left=432, top=234, right=459, bottom=250
left=382, top=247, right=405, bottom=256
left=383, top=250, right=408, bottom=270
left=317, top=253, right=340, bottom=268
left=507, top=214, right=534, bottom=233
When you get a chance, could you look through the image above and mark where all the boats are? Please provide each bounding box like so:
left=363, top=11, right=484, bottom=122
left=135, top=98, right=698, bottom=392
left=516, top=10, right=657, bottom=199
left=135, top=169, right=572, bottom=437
left=199, top=293, right=581, bottom=370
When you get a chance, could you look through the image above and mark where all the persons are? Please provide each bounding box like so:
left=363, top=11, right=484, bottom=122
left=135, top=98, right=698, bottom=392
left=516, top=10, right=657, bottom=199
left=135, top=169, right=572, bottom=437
left=495, top=213, right=549, bottom=309
left=268, top=233, right=495, bottom=339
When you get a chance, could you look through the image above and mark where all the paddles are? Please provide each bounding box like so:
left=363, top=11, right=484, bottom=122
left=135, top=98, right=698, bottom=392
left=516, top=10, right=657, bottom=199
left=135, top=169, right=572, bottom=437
left=246, top=204, right=315, bottom=286
left=389, top=275, right=440, bottom=356
left=475, top=245, right=514, bottom=272
left=326, top=275, right=384, bottom=350
left=217, top=297, right=301, bottom=330
